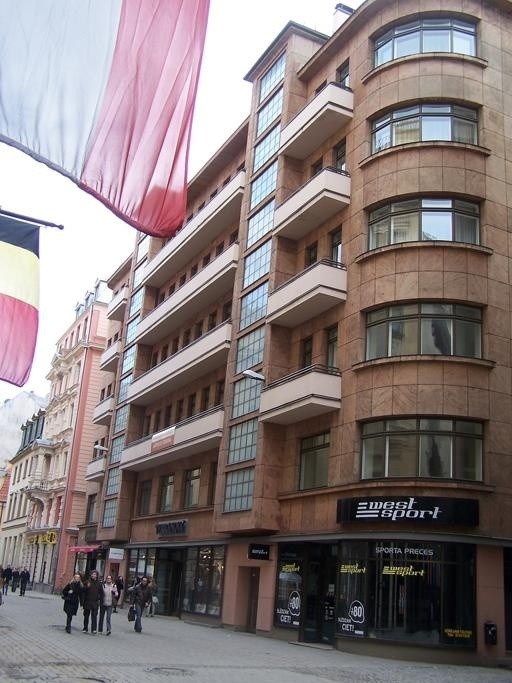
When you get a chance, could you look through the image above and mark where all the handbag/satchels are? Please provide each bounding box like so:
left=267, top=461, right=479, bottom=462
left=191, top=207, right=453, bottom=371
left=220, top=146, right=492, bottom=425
left=127, top=606, right=135, bottom=622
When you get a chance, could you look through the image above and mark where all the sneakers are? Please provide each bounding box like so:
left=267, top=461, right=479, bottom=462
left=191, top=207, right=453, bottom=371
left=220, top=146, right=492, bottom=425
left=83, top=629, right=103, bottom=635
left=66, top=627, right=71, bottom=633
left=134, top=626, right=141, bottom=633
left=106, top=630, right=112, bottom=636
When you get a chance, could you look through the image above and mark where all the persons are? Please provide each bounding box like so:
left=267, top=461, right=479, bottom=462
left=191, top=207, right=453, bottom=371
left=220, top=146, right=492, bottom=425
left=0, top=562, right=30, bottom=605
left=129, top=576, right=153, bottom=632
left=98, top=575, right=120, bottom=635
left=81, top=569, right=102, bottom=633
left=77, top=570, right=158, bottom=616
left=62, top=573, right=82, bottom=633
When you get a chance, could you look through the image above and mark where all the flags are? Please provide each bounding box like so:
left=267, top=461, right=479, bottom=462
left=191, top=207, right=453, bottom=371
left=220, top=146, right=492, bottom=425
left=0, top=213, right=43, bottom=389
left=1, top=0, right=212, bottom=239
